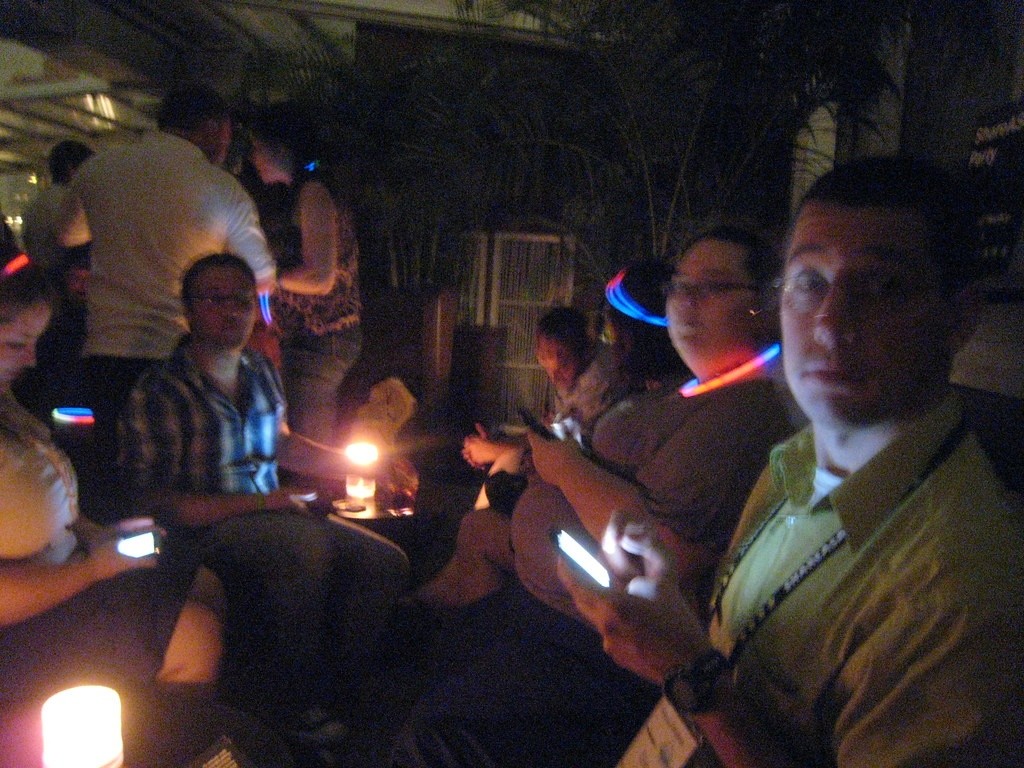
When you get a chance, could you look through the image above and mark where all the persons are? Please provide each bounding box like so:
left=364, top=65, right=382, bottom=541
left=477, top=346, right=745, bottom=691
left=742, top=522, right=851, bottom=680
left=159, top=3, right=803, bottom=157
left=5, top=82, right=367, bottom=527
left=117, top=249, right=412, bottom=768
left=556, top=155, right=1024, bottom=767
left=0, top=266, right=254, bottom=767
left=406, top=223, right=814, bottom=768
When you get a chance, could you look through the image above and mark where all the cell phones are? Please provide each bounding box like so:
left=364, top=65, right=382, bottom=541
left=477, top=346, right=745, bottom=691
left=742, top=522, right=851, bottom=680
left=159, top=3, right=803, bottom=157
left=520, top=411, right=558, bottom=442
left=118, top=529, right=160, bottom=558
left=551, top=529, right=610, bottom=591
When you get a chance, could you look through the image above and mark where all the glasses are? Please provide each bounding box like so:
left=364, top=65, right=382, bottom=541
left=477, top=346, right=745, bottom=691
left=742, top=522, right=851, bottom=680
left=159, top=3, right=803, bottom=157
left=195, top=294, right=253, bottom=308
left=775, top=274, right=946, bottom=315
left=662, top=280, right=763, bottom=299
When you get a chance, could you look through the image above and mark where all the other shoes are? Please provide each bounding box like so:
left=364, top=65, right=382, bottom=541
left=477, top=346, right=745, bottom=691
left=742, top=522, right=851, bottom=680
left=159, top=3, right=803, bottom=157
left=263, top=700, right=348, bottom=747
left=416, top=558, right=503, bottom=608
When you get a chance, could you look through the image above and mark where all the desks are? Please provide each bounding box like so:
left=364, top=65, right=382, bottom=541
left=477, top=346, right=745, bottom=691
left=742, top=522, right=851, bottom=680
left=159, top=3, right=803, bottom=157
left=0, top=690, right=299, bottom=768
left=330, top=483, right=433, bottom=524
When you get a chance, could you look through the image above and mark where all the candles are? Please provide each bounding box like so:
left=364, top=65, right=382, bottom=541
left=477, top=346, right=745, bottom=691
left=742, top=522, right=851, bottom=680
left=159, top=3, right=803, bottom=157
left=348, top=443, right=378, bottom=497
left=42, top=685, right=124, bottom=768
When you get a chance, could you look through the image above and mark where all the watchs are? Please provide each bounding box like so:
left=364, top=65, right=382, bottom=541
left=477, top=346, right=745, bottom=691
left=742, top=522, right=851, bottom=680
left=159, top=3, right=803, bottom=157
left=664, top=647, right=730, bottom=715
